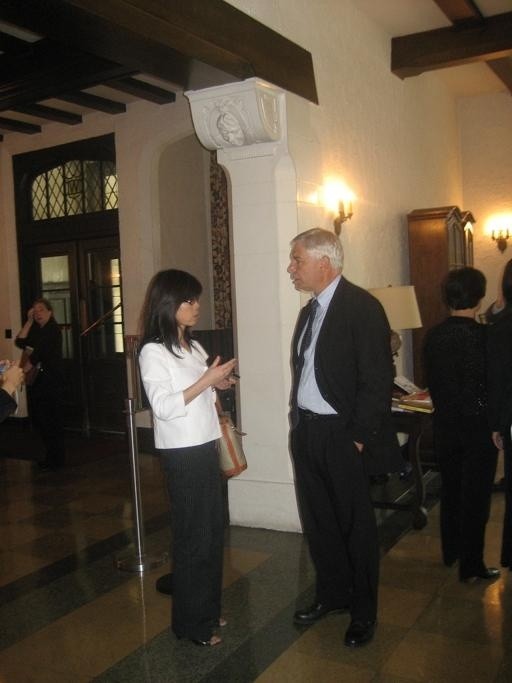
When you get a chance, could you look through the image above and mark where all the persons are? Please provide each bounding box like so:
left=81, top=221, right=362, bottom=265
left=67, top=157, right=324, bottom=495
left=486, top=257, right=512, bottom=575
left=216, top=113, right=245, bottom=147
left=484, top=271, right=509, bottom=323
left=417, top=265, right=503, bottom=587
left=0, top=355, right=27, bottom=426
left=282, top=224, right=398, bottom=649
left=13, top=296, right=70, bottom=472
left=133, top=264, right=243, bottom=649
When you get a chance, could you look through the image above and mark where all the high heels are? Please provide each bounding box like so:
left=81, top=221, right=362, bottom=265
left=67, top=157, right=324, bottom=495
left=456, top=563, right=502, bottom=583
left=174, top=630, right=222, bottom=647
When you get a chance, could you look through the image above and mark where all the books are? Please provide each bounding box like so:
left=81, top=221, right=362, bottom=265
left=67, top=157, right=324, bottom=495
left=389, top=373, right=438, bottom=416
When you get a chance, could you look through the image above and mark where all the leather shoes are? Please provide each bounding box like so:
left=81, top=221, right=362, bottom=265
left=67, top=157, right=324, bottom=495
left=291, top=596, right=349, bottom=623
left=342, top=616, right=379, bottom=648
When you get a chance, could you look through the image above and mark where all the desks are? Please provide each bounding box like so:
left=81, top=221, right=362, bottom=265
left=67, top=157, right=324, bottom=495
left=370, top=406, right=442, bottom=530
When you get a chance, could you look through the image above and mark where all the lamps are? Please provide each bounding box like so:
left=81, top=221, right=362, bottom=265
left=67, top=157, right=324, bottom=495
left=366, top=283, right=424, bottom=379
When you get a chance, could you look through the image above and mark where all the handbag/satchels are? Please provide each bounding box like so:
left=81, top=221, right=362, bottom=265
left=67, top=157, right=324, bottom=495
left=211, top=394, right=248, bottom=478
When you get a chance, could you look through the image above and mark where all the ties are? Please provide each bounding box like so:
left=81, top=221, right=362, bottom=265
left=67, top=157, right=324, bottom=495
left=289, top=301, right=318, bottom=433
